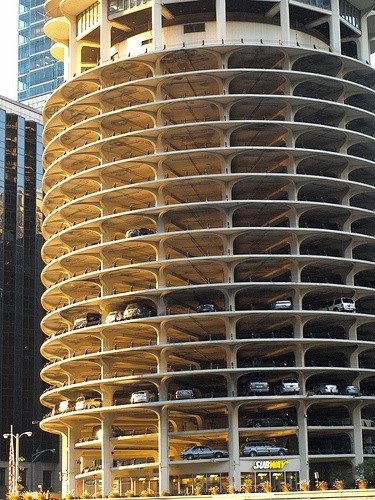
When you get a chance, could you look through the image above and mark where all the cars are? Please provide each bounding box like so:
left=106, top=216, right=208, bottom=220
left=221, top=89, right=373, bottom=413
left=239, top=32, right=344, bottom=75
left=126, top=228, right=154, bottom=238
left=338, top=384, right=359, bottom=396
left=196, top=300, right=220, bottom=312
left=129, top=390, right=156, bottom=403
left=104, top=310, right=123, bottom=323
left=180, top=445, right=229, bottom=460
left=312, top=382, right=338, bottom=395
left=56, top=396, right=103, bottom=409
left=170, top=387, right=194, bottom=400
left=270, top=299, right=292, bottom=310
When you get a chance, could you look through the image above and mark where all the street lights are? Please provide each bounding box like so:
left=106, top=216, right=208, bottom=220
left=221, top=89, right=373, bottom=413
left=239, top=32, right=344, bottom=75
left=3, top=431, right=33, bottom=495
left=30, top=447, right=55, bottom=491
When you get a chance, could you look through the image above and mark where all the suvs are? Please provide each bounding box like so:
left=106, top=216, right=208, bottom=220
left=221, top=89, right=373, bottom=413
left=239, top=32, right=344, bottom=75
left=241, top=441, right=288, bottom=457
left=92, top=425, right=124, bottom=439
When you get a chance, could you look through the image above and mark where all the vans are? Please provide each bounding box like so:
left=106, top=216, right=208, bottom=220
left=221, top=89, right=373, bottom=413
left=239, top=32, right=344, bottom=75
left=244, top=409, right=293, bottom=427
left=124, top=303, right=150, bottom=320
left=325, top=297, right=355, bottom=313
left=271, top=377, right=300, bottom=395
left=240, top=378, right=270, bottom=396
left=74, top=313, right=102, bottom=328
